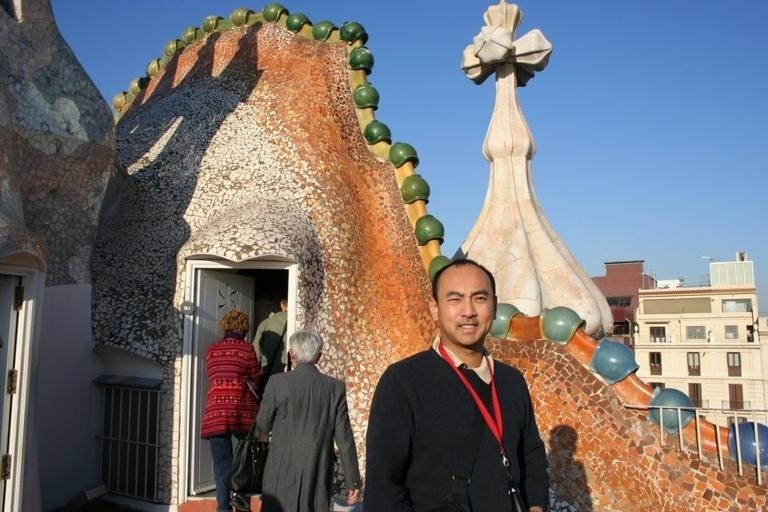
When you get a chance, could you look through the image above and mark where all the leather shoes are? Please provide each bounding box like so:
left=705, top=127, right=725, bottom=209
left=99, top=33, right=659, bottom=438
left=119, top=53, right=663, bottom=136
left=228, top=493, right=250, bottom=512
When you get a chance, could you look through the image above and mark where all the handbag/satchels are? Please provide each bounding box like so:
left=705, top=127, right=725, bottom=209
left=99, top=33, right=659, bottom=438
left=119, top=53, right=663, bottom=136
left=226, top=430, right=269, bottom=493
left=414, top=473, right=473, bottom=512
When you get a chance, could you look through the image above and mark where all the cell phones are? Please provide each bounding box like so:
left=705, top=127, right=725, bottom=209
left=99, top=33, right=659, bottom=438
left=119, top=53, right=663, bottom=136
left=507, top=482, right=526, bottom=512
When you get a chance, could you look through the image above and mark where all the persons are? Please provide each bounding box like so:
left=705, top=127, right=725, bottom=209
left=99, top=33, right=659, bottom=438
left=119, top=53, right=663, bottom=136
left=362, top=258, right=548, bottom=512
left=252, top=297, right=287, bottom=372
left=253, top=329, right=362, bottom=512
left=201, top=309, right=264, bottom=511
